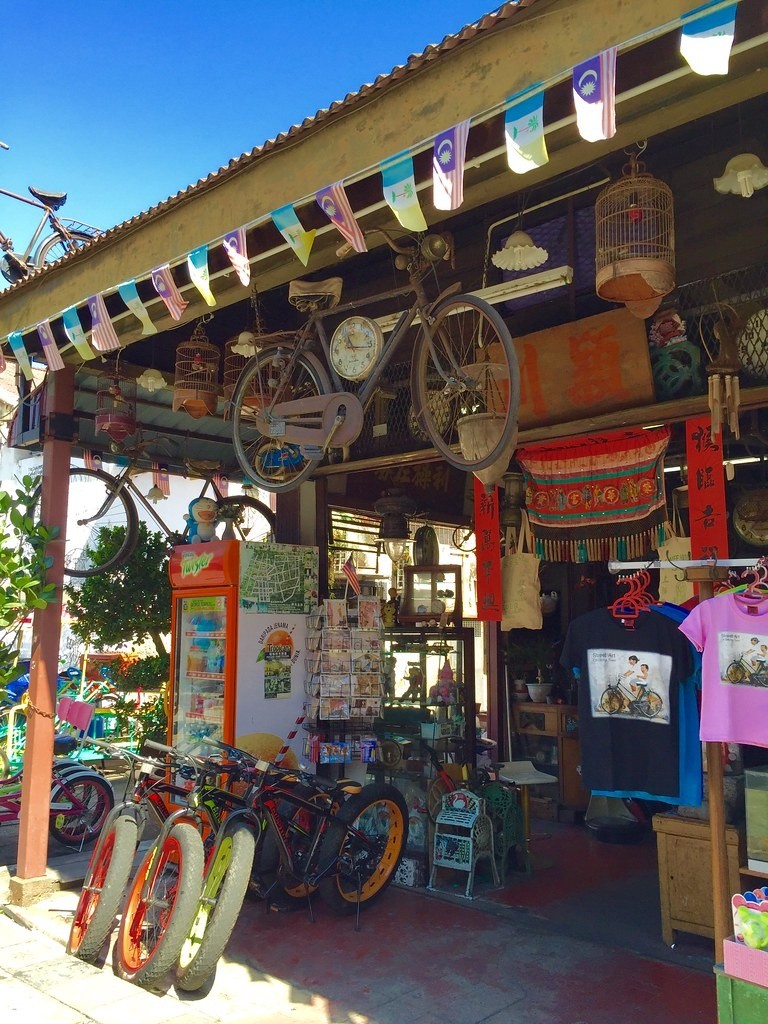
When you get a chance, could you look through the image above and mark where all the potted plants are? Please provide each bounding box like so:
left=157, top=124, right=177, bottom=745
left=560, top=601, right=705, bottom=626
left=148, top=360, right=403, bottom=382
left=527, top=659, right=552, bottom=702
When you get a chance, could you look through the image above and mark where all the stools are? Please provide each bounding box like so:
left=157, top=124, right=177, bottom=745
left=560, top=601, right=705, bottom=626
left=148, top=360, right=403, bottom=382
left=496, top=761, right=559, bottom=851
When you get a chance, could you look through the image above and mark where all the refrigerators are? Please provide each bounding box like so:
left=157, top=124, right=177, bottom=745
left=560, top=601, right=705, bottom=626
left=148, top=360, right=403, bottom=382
left=164, top=541, right=315, bottom=867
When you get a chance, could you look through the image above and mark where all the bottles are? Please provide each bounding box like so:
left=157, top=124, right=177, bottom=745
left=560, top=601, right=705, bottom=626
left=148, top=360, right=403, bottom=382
left=188, top=612, right=225, bottom=632
left=178, top=775, right=213, bottom=811
left=184, top=724, right=222, bottom=758
left=187, top=640, right=224, bottom=673
left=186, top=693, right=224, bottom=724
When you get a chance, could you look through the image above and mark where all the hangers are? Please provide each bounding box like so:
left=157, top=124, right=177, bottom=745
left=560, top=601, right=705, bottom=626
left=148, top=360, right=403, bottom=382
left=607, top=569, right=662, bottom=632
left=734, top=557, right=768, bottom=601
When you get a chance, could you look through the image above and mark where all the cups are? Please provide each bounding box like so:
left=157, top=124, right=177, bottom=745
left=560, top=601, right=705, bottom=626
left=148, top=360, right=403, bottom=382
left=514, top=680, right=525, bottom=693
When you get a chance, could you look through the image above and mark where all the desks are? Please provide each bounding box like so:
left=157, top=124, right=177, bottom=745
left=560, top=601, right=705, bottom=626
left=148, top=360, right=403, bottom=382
left=708, top=965, right=768, bottom=1024
left=649, top=812, right=751, bottom=950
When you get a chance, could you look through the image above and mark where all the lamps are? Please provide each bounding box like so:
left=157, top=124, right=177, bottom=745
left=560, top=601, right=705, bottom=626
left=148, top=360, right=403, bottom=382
left=135, top=364, right=168, bottom=390
left=491, top=201, right=548, bottom=270
left=714, top=138, right=768, bottom=199
left=375, top=485, right=427, bottom=563
left=231, top=324, right=259, bottom=359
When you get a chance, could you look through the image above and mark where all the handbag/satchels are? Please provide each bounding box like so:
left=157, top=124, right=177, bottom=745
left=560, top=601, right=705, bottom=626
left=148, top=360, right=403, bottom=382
left=500, top=508, right=543, bottom=631
left=655, top=492, right=694, bottom=604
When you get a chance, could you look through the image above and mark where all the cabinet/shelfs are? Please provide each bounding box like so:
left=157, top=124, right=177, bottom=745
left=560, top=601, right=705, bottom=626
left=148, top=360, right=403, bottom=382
left=513, top=700, right=580, bottom=809
left=368, top=625, right=478, bottom=780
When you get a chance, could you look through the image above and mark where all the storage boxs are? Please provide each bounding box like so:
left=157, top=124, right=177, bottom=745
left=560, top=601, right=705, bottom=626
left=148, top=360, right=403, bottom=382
left=723, top=935, right=768, bottom=987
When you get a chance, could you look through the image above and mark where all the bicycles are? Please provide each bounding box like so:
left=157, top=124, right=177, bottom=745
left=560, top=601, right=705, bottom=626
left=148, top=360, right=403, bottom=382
left=224, top=228, right=523, bottom=492
left=0, top=137, right=101, bottom=284
left=1, top=720, right=411, bottom=998
left=22, top=431, right=279, bottom=578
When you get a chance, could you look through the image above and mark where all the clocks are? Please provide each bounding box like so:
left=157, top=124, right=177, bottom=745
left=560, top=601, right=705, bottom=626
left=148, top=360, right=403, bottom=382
left=405, top=390, right=454, bottom=442
left=329, top=315, right=382, bottom=376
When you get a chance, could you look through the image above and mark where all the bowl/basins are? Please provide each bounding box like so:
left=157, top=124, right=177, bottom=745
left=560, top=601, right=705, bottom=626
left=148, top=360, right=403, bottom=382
left=511, top=693, right=529, bottom=701
